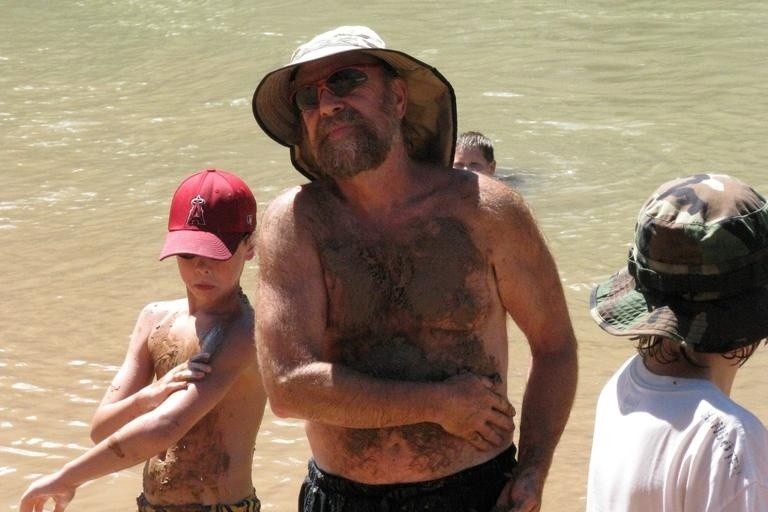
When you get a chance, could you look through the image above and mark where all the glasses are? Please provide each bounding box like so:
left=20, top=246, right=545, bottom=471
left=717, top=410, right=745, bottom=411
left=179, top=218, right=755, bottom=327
left=288, top=63, right=384, bottom=111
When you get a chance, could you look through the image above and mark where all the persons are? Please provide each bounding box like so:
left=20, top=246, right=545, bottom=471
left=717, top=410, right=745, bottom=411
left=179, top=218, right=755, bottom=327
left=21, top=168, right=268, bottom=512
left=253, top=24, right=578, bottom=512
left=453, top=132, right=496, bottom=177
left=584, top=172, right=768, bottom=512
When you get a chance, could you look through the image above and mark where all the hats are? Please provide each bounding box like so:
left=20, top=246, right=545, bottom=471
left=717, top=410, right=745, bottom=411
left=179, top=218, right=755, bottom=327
left=589, top=174, right=768, bottom=353
left=159, top=169, right=256, bottom=260
left=252, top=25, right=457, bottom=182
